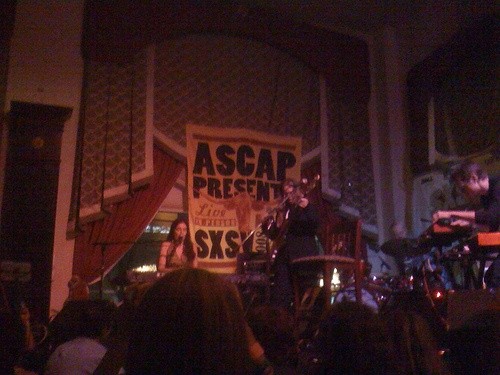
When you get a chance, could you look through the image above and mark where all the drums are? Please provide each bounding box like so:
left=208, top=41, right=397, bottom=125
left=332, top=282, right=390, bottom=313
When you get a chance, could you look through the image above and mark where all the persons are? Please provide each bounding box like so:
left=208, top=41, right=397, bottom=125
left=432, top=161, right=500, bottom=230
left=157, top=217, right=198, bottom=275
left=0, top=268, right=500, bottom=375
left=261, top=182, right=320, bottom=308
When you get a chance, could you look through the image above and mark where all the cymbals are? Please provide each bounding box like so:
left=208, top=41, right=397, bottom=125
left=380, top=237, right=431, bottom=256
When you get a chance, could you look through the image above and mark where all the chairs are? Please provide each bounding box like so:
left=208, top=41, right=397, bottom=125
left=291, top=218, right=363, bottom=333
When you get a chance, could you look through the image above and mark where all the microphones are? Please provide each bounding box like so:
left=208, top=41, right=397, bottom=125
left=175, top=234, right=183, bottom=246
left=378, top=255, right=391, bottom=269
